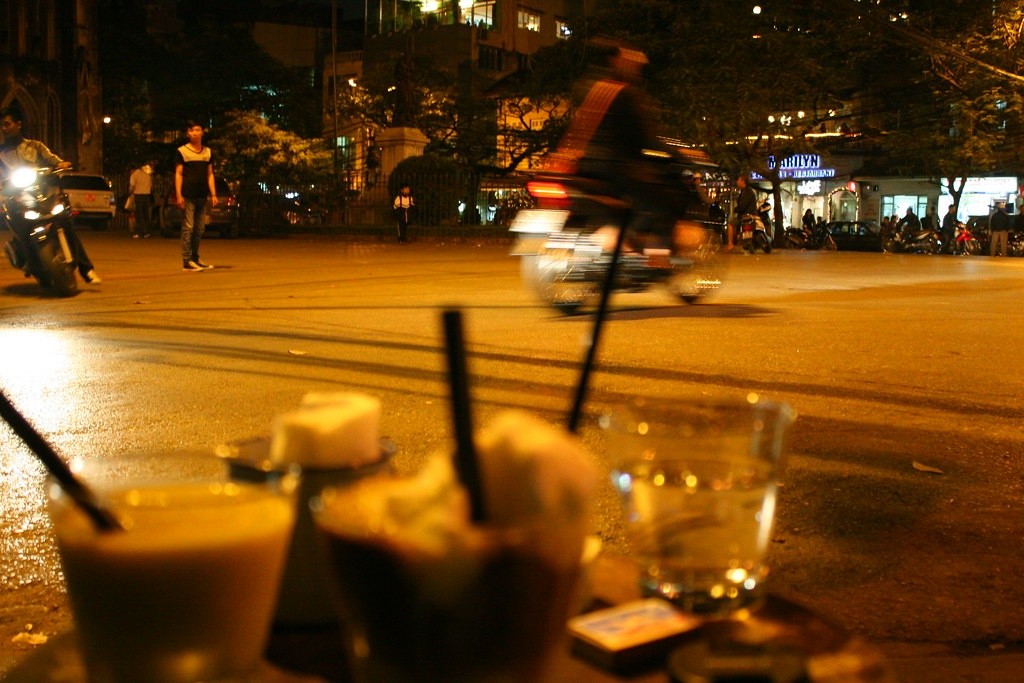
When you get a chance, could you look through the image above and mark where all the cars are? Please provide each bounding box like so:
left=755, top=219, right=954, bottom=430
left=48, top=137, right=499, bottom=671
left=826, top=220, right=897, bottom=252
left=158, top=171, right=241, bottom=240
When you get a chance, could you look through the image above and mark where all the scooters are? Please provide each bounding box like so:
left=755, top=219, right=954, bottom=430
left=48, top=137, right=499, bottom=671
left=733, top=196, right=776, bottom=254
left=512, top=151, right=732, bottom=315
left=936, top=222, right=1024, bottom=257
left=0, top=163, right=84, bottom=299
left=787, top=218, right=838, bottom=251
left=883, top=222, right=938, bottom=255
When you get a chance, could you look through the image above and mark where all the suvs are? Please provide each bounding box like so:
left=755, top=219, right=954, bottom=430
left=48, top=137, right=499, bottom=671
left=58, top=171, right=116, bottom=232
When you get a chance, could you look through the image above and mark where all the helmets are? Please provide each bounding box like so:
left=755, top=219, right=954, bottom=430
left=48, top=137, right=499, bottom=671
left=682, top=169, right=693, bottom=176
left=997, top=202, right=1005, bottom=208
left=693, top=172, right=702, bottom=178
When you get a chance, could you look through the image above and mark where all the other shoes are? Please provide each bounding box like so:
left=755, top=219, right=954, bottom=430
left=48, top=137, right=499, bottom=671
left=144, top=234, right=150, bottom=238
left=133, top=234, right=138, bottom=238
left=82, top=270, right=102, bottom=284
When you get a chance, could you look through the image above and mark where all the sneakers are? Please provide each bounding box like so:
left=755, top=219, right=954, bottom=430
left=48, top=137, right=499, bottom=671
left=183, top=260, right=205, bottom=271
left=195, top=261, right=214, bottom=269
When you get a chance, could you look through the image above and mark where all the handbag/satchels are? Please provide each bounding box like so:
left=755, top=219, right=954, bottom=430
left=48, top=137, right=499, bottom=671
left=125, top=194, right=134, bottom=209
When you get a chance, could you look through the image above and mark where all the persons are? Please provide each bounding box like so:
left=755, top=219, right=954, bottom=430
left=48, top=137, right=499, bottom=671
left=802, top=209, right=816, bottom=234
left=127, top=165, right=152, bottom=239
left=709, top=196, right=725, bottom=243
left=899, top=207, right=921, bottom=246
left=393, top=184, right=416, bottom=244
left=722, top=176, right=757, bottom=251
left=939, top=204, right=963, bottom=254
left=545, top=40, right=698, bottom=265
left=881, top=217, right=893, bottom=253
left=926, top=206, right=941, bottom=231
left=990, top=203, right=1010, bottom=256
left=889, top=215, right=898, bottom=238
left=1014, top=205, right=1024, bottom=231
left=174, top=120, right=218, bottom=272
left=0, top=106, right=101, bottom=285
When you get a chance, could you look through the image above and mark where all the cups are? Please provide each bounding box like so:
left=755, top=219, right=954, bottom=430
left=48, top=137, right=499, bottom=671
left=308, top=472, right=596, bottom=682
left=45, top=454, right=300, bottom=683
left=214, top=430, right=398, bottom=682
left=599, top=397, right=791, bottom=624
